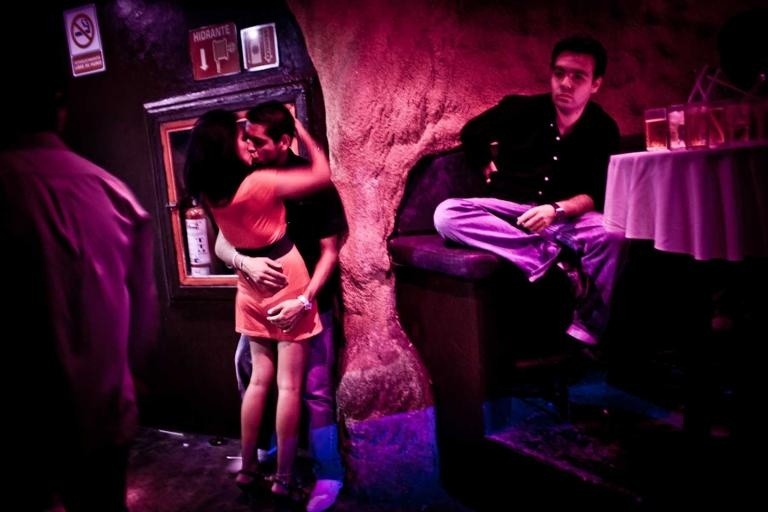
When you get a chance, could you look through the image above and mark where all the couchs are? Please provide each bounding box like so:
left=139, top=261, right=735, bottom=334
left=388, top=153, right=561, bottom=417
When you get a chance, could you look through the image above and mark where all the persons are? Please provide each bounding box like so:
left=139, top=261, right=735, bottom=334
left=432, top=33, right=628, bottom=370
left=0, top=57, right=163, bottom=511
left=182, top=102, right=331, bottom=512
left=211, top=100, right=350, bottom=512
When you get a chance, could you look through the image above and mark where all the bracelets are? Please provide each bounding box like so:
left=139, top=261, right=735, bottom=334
left=231, top=251, right=249, bottom=272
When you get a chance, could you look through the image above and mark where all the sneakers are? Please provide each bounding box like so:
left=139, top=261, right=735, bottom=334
left=224, top=447, right=270, bottom=473
left=306, top=479, right=344, bottom=512
left=567, top=267, right=590, bottom=299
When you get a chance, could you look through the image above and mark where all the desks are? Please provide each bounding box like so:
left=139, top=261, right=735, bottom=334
left=603, top=143, right=768, bottom=261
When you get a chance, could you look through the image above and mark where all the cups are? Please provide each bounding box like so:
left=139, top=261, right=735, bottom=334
left=642, top=100, right=767, bottom=153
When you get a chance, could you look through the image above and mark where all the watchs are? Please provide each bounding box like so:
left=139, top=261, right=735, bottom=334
left=296, top=294, right=312, bottom=311
left=550, top=202, right=565, bottom=221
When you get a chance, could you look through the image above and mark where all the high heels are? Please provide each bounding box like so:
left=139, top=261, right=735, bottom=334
left=234, top=469, right=264, bottom=496
left=270, top=480, right=303, bottom=512
